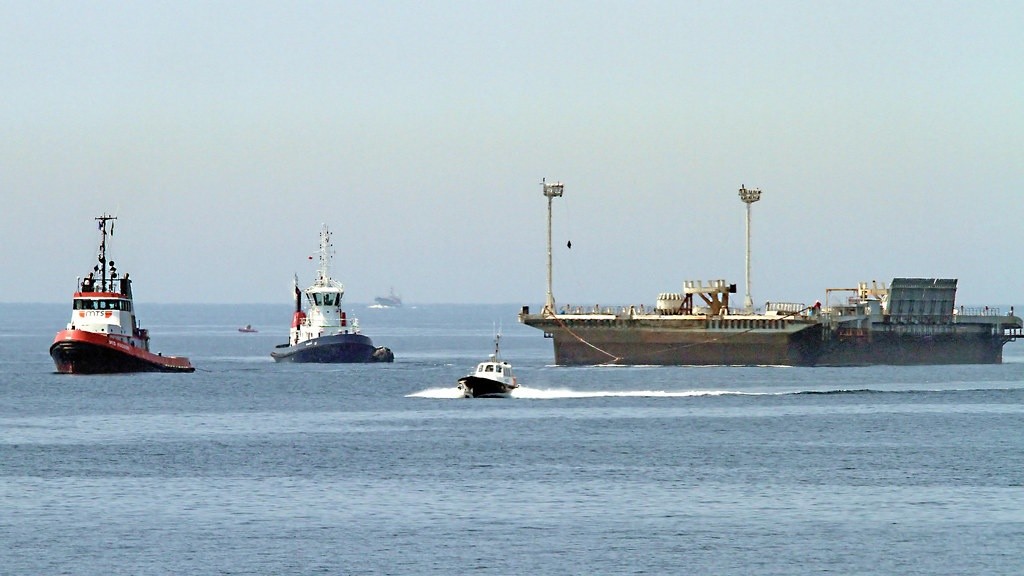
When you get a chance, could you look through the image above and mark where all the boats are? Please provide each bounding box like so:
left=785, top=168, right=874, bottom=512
left=49, top=210, right=196, bottom=373
left=270, top=219, right=395, bottom=365
left=372, top=287, right=402, bottom=307
left=519, top=178, right=1024, bottom=367
left=458, top=319, right=520, bottom=400
left=239, top=324, right=258, bottom=333
